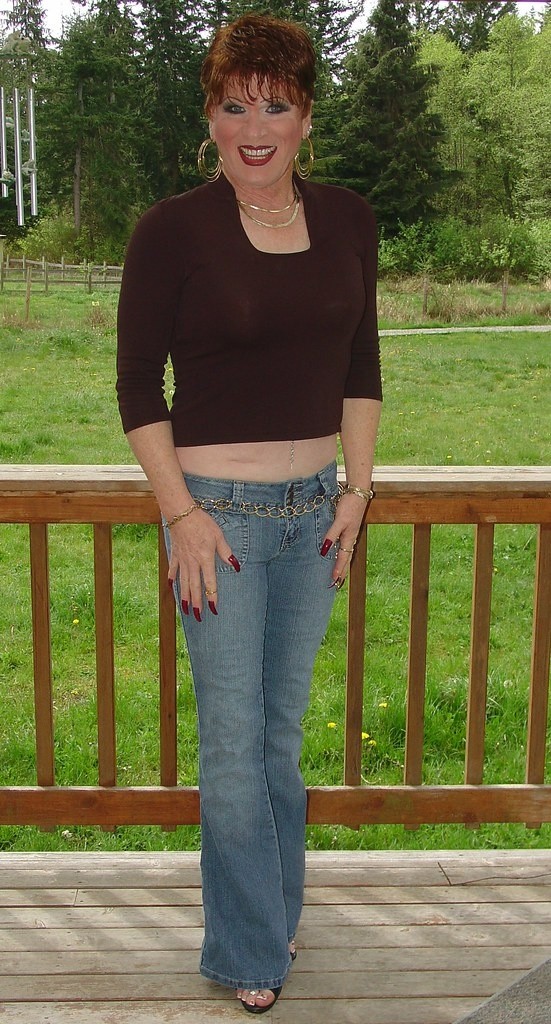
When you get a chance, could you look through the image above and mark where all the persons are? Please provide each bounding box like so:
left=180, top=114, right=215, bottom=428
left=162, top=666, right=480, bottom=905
left=115, top=14, right=384, bottom=1014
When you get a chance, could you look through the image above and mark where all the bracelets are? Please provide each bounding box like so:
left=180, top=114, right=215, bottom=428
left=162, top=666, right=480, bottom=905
left=343, top=484, right=373, bottom=503
left=165, top=504, right=202, bottom=528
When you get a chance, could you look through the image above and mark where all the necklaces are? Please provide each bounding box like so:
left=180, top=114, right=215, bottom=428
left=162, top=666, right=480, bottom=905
left=236, top=193, right=300, bottom=228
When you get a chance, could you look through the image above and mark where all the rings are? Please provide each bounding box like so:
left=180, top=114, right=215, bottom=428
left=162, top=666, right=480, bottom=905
left=205, top=590, right=216, bottom=595
left=339, top=547, right=352, bottom=553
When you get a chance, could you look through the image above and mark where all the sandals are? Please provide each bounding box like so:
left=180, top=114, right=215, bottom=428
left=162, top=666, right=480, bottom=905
left=237, top=985, right=282, bottom=1013
left=288, top=941, right=297, bottom=960
